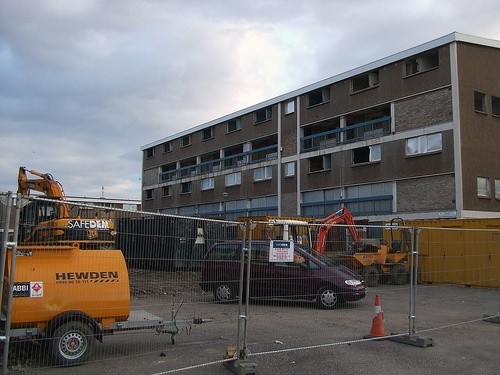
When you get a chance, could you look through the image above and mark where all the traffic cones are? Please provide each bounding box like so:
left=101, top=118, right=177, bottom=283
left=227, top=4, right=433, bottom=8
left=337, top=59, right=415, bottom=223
left=362, top=294, right=389, bottom=341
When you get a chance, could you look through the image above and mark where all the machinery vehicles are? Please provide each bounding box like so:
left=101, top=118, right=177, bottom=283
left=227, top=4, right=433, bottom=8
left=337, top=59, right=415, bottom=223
left=0, top=166, right=130, bottom=368
left=317, top=206, right=410, bottom=287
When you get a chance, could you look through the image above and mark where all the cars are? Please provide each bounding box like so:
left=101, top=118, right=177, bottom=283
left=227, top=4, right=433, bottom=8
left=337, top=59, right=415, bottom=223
left=199, top=240, right=367, bottom=310
